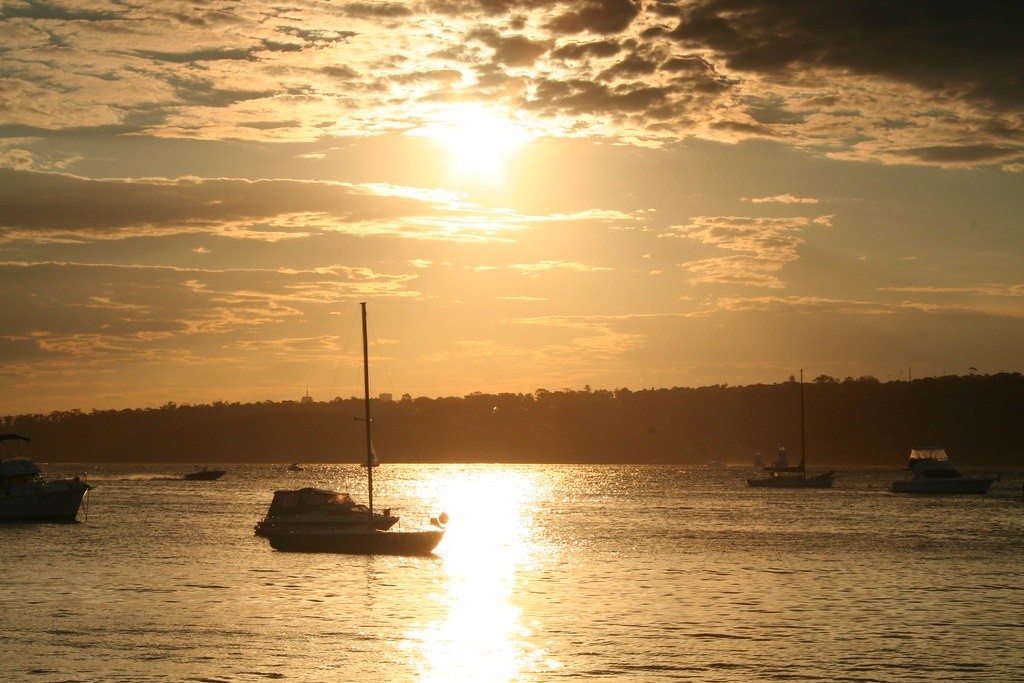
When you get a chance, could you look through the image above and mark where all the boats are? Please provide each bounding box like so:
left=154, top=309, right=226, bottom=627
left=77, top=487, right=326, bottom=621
left=892, top=447, right=993, bottom=495
left=0, top=435, right=95, bottom=524
left=288, top=464, right=303, bottom=471
left=253, top=488, right=400, bottom=539
left=184, top=470, right=226, bottom=480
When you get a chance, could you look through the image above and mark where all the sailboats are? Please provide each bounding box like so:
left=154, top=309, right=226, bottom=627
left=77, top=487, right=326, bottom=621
left=746, top=370, right=836, bottom=488
left=361, top=435, right=378, bottom=467
left=270, top=301, right=448, bottom=557
